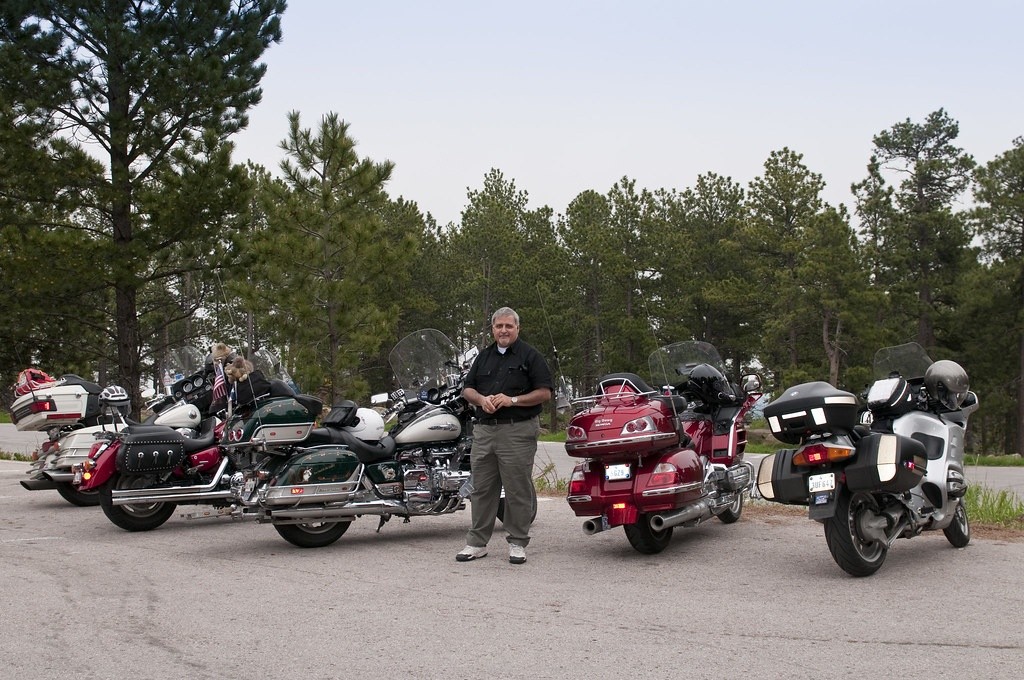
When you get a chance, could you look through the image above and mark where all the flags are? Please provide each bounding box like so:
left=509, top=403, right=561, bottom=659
left=213, top=364, right=227, bottom=401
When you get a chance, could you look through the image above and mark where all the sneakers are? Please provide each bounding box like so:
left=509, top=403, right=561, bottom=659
left=507, top=542, right=527, bottom=564
left=455, top=545, right=489, bottom=562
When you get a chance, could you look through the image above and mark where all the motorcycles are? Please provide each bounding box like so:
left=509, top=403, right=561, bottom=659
left=9, top=344, right=228, bottom=506
left=71, top=350, right=302, bottom=533
left=216, top=328, right=538, bottom=550
left=753, top=342, right=980, bottom=577
left=564, top=341, right=763, bottom=554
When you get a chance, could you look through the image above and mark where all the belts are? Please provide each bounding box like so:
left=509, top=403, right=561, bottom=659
left=472, top=415, right=539, bottom=427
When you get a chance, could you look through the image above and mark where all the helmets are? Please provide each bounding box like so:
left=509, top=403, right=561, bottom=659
left=344, top=406, right=384, bottom=441
left=688, top=363, right=725, bottom=402
left=98, top=384, right=130, bottom=407
left=924, top=359, right=970, bottom=400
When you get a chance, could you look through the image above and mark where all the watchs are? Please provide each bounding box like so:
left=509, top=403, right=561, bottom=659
left=511, top=396, right=518, bottom=406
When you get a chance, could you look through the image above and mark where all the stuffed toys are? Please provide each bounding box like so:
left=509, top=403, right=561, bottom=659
left=211, top=341, right=249, bottom=384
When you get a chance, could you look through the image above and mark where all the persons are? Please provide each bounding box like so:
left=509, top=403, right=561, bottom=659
left=455, top=307, right=555, bottom=565
